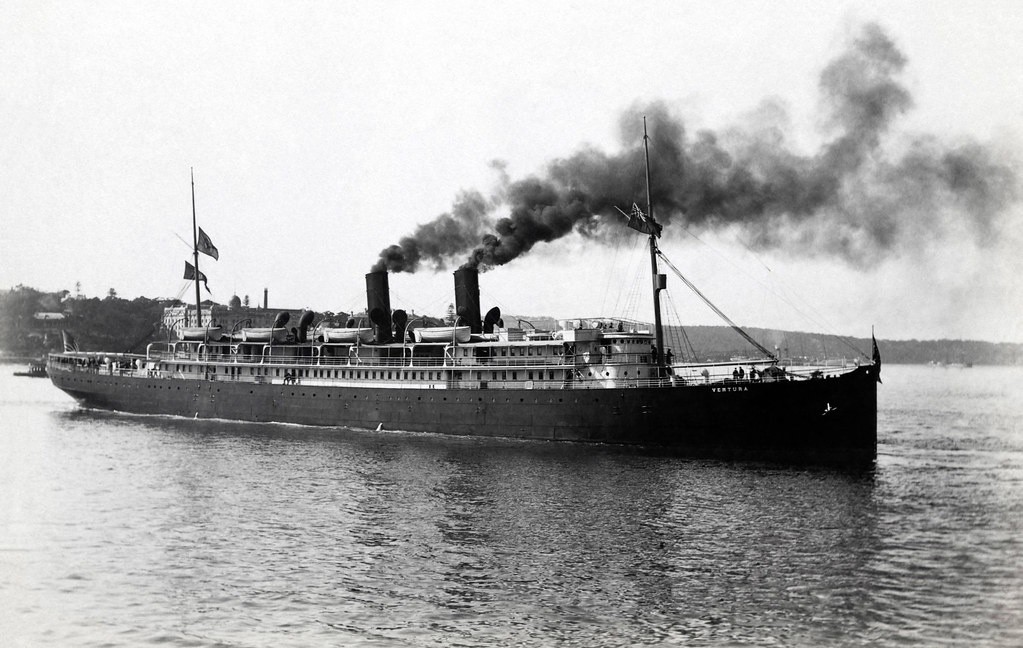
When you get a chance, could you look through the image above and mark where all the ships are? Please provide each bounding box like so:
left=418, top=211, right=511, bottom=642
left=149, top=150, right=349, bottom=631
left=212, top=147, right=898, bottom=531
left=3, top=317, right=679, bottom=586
left=44, top=112, right=885, bottom=477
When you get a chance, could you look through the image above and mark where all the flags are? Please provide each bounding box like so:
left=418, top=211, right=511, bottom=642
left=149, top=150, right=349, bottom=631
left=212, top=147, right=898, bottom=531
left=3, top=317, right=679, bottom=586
left=62, top=329, right=79, bottom=351
left=197, top=225, right=218, bottom=260
left=184, top=260, right=211, bottom=295
left=628, top=201, right=663, bottom=239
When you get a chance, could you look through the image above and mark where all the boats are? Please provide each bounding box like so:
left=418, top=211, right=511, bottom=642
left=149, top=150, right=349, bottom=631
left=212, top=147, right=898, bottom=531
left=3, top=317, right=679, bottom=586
left=13, top=359, right=49, bottom=378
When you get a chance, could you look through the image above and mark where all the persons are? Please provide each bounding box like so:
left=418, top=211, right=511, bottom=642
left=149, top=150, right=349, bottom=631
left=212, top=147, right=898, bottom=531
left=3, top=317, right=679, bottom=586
left=578, top=322, right=623, bottom=331
left=283, top=373, right=296, bottom=385
left=734, top=367, right=744, bottom=379
left=651, top=346, right=673, bottom=364
